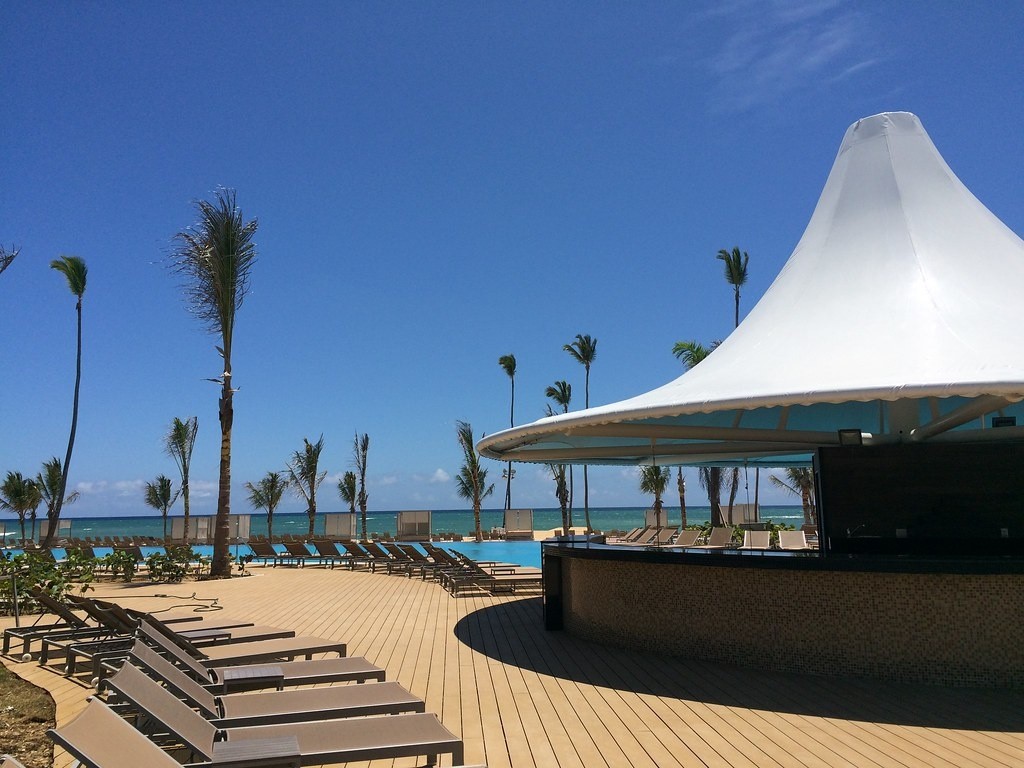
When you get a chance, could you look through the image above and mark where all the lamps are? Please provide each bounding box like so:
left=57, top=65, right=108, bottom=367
left=838, top=428, right=863, bottom=446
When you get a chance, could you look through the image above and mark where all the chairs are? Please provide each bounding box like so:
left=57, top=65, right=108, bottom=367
left=0, top=528, right=812, bottom=768
